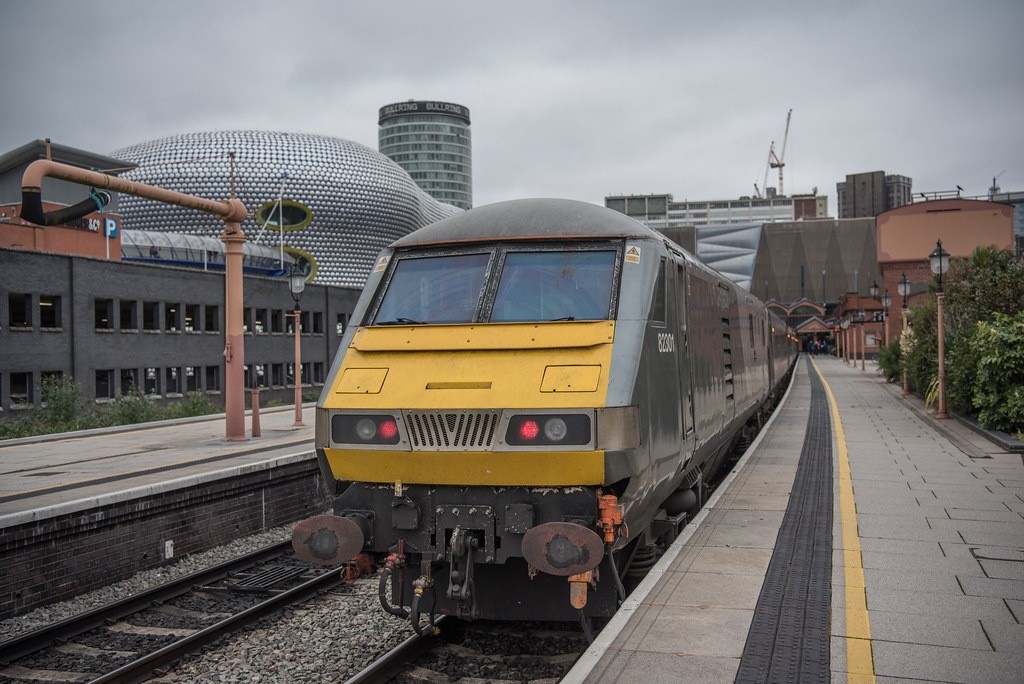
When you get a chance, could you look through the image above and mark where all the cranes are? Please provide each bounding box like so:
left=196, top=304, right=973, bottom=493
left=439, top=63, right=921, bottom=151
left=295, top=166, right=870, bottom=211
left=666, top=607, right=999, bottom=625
left=760, top=108, right=793, bottom=199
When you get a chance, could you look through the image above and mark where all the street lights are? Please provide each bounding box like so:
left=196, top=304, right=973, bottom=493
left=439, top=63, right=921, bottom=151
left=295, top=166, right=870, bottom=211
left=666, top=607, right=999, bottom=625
left=897, top=273, right=912, bottom=396
left=858, top=309, right=866, bottom=370
left=880, top=289, right=892, bottom=383
left=288, top=262, right=306, bottom=426
left=928, top=236, right=951, bottom=419
left=834, top=311, right=858, bottom=369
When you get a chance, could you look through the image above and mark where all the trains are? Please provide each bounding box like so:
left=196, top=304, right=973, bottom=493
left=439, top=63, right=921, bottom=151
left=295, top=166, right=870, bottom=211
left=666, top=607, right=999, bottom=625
left=290, top=197, right=800, bottom=644
